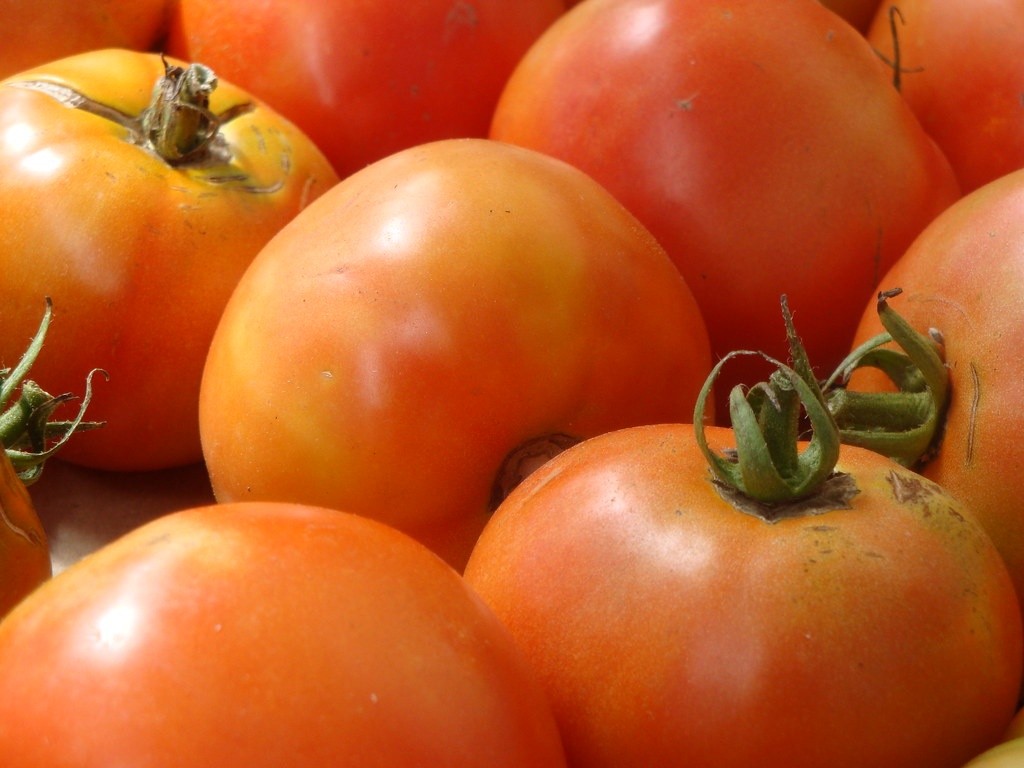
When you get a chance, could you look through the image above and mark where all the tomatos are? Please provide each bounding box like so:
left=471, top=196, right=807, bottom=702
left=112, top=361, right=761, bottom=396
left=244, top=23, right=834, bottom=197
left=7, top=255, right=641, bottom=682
left=0, top=0, right=1024, bottom=768
